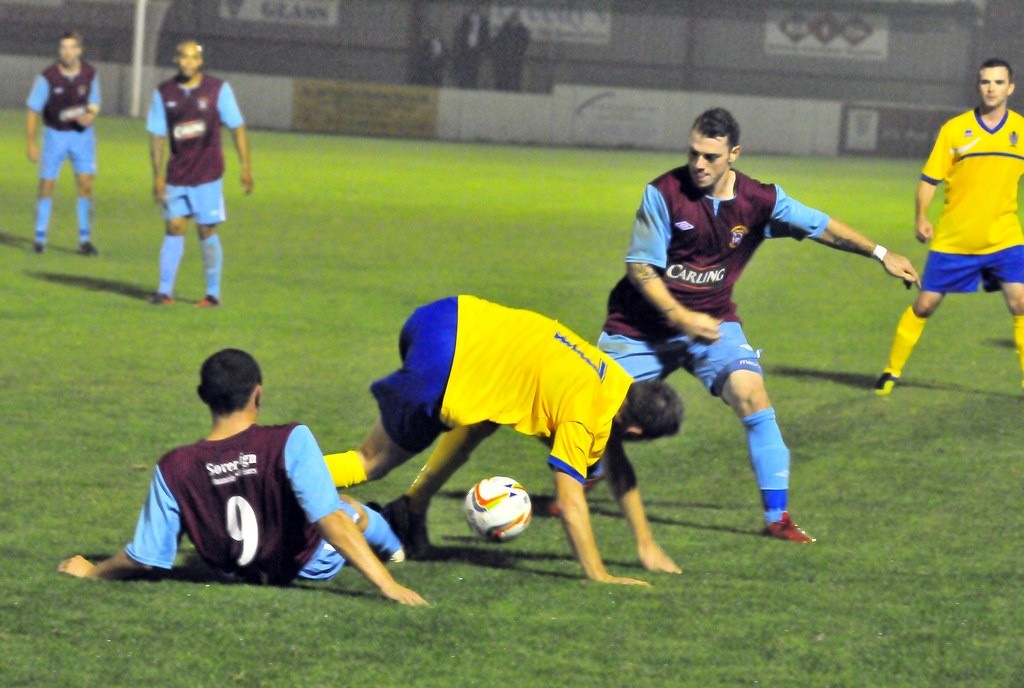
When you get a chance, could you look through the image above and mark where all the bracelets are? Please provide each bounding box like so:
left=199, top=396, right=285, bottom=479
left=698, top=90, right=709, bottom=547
left=872, top=244, right=887, bottom=262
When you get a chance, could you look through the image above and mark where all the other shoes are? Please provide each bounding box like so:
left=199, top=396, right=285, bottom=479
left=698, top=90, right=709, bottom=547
left=196, top=297, right=221, bottom=309
left=78, top=243, right=98, bottom=254
left=35, top=243, right=44, bottom=252
left=874, top=372, right=895, bottom=395
left=154, top=294, right=175, bottom=306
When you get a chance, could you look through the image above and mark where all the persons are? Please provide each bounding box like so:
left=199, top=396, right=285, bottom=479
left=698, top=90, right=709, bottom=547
left=26, top=30, right=99, bottom=257
left=415, top=8, right=530, bottom=90
left=320, top=296, right=684, bottom=590
left=872, top=59, right=1024, bottom=395
left=547, top=107, right=923, bottom=547
left=55, top=347, right=426, bottom=604
left=144, top=38, right=254, bottom=308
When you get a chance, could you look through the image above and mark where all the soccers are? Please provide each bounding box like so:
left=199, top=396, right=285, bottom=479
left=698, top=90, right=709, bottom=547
left=462, top=475, right=533, bottom=543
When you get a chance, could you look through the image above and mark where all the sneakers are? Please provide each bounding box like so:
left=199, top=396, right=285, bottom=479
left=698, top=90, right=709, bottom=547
left=381, top=496, right=431, bottom=559
left=763, top=511, right=814, bottom=543
left=548, top=481, right=595, bottom=516
